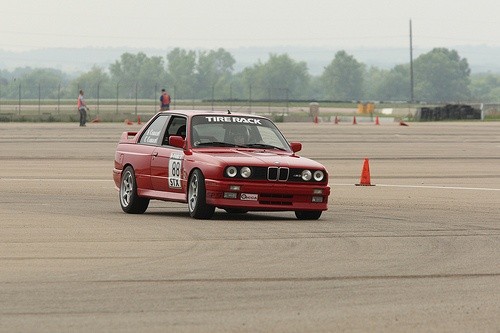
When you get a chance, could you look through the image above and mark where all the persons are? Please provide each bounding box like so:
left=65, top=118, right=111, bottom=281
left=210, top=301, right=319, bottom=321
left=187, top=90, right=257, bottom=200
left=224, top=126, right=249, bottom=144
left=77, top=90, right=89, bottom=126
left=160, top=89, right=170, bottom=111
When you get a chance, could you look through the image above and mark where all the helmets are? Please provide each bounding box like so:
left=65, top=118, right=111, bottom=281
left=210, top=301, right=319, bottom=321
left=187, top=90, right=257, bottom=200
left=229, top=127, right=249, bottom=142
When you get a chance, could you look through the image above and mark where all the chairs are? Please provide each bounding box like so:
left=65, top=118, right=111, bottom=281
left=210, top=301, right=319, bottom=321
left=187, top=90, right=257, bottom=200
left=175, top=124, right=201, bottom=141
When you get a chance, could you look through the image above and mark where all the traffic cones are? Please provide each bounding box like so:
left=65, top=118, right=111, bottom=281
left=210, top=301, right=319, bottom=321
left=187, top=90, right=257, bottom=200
left=375, top=115, right=379, bottom=125
left=400, top=121, right=408, bottom=126
left=314, top=114, right=318, bottom=123
left=354, top=159, right=376, bottom=186
left=136, top=115, right=141, bottom=125
left=353, top=116, right=357, bottom=125
left=334, top=115, right=338, bottom=124
left=124, top=120, right=134, bottom=125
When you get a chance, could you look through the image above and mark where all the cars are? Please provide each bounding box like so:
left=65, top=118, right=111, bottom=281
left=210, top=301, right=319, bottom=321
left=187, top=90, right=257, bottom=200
left=111, top=109, right=331, bottom=220
